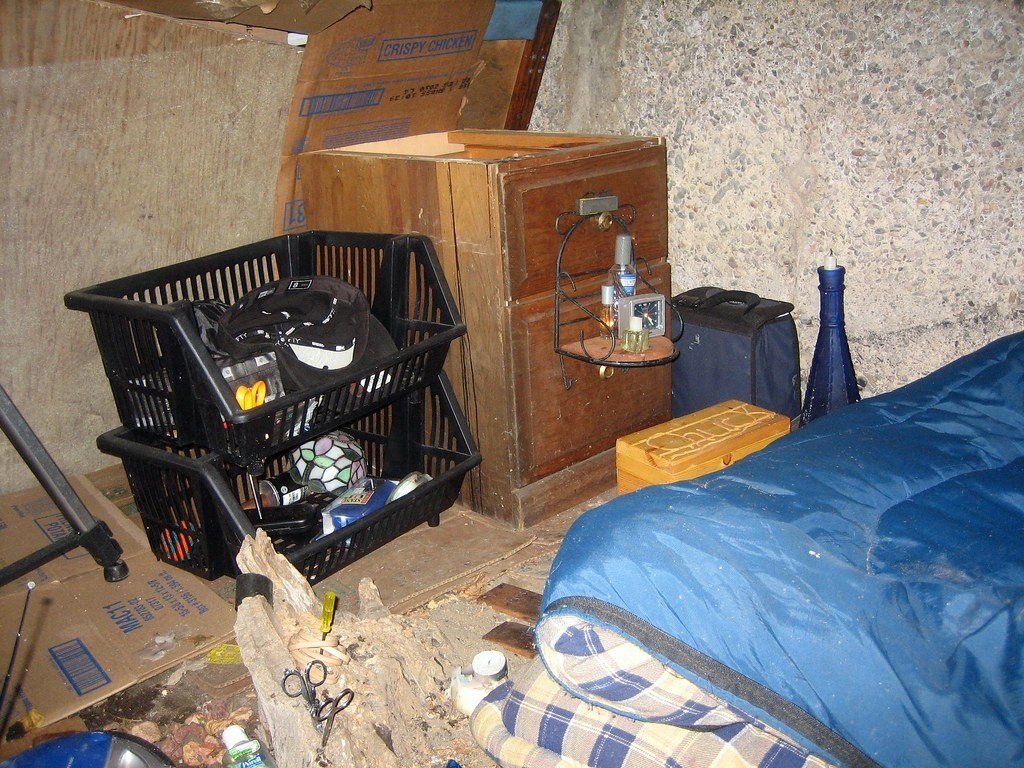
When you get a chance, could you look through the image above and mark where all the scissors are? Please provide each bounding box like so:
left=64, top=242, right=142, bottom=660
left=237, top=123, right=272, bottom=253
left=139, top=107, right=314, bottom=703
left=235, top=380, right=267, bottom=411
left=308, top=689, right=355, bottom=748
left=281, top=660, right=329, bottom=711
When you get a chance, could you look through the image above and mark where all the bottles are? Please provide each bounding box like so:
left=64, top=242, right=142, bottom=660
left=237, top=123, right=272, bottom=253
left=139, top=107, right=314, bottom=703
left=795, top=264, right=862, bottom=431
left=222, top=724, right=276, bottom=768
left=620, top=317, right=651, bottom=354
left=600, top=284, right=617, bottom=337
left=608, top=234, right=636, bottom=297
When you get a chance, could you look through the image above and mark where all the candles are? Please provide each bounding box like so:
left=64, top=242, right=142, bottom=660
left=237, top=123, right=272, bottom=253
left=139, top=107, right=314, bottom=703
left=823, top=249, right=837, bottom=270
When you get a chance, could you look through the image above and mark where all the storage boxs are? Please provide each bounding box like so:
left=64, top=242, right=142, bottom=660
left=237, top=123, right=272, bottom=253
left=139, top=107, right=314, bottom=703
left=616, top=400, right=791, bottom=497
left=61, top=229, right=482, bottom=593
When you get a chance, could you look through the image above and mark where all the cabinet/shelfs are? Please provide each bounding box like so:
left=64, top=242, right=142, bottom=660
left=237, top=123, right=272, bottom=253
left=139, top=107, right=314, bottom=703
left=299, top=128, right=674, bottom=530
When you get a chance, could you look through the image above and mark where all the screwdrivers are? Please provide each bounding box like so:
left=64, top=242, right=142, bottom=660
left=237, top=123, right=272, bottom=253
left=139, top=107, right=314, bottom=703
left=319, top=590, right=337, bottom=656
